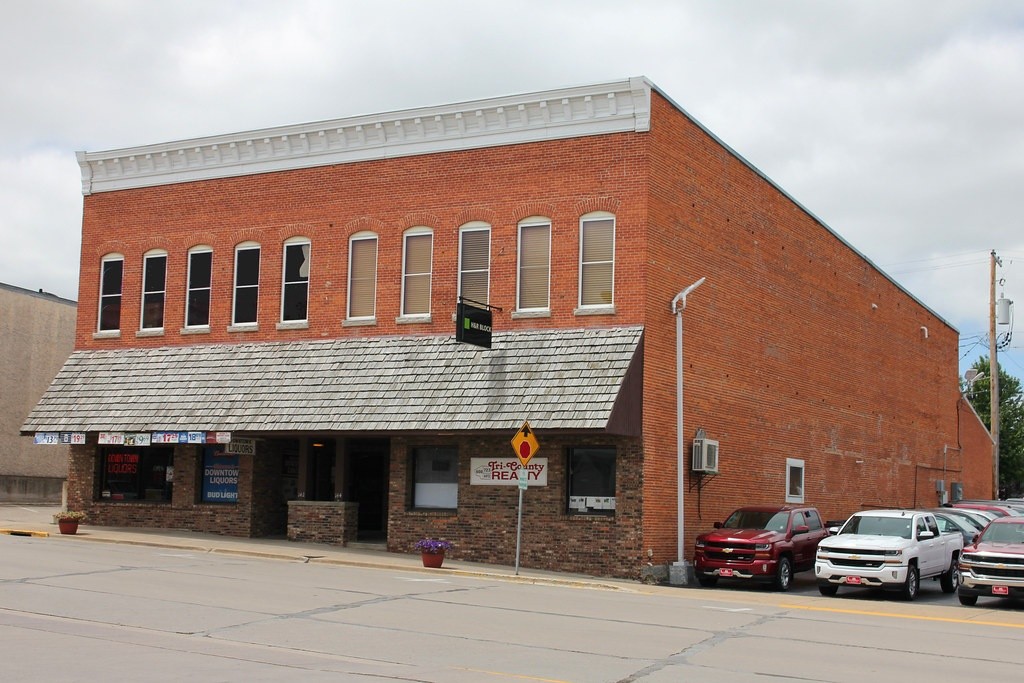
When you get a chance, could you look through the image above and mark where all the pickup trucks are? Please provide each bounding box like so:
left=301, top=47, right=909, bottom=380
left=692, top=506, right=837, bottom=592
left=959, top=515, right=1024, bottom=603
left=813, top=508, right=965, bottom=600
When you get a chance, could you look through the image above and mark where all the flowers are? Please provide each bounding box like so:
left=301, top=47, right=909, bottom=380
left=54, top=511, right=89, bottom=523
left=417, top=539, right=454, bottom=553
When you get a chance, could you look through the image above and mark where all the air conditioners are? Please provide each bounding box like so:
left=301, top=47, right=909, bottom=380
left=692, top=438, right=719, bottom=472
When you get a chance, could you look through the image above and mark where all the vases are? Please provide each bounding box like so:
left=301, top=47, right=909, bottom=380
left=421, top=553, right=444, bottom=567
left=58, top=520, right=79, bottom=534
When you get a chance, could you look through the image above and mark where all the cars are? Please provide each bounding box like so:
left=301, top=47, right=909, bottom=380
left=931, top=496, right=1024, bottom=576
